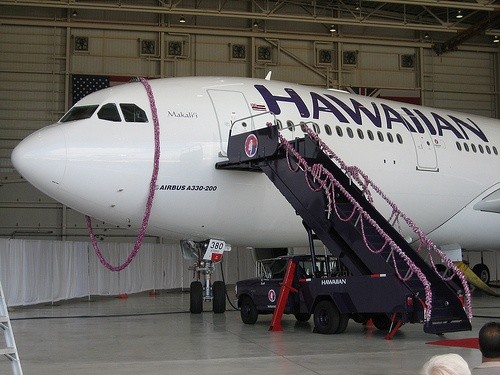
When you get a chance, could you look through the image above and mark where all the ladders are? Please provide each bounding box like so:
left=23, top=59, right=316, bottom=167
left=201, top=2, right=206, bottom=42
left=255, top=135, right=473, bottom=333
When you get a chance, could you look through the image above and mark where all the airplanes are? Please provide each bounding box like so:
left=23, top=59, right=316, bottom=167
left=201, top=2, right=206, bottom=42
left=9, top=70, right=500, bottom=313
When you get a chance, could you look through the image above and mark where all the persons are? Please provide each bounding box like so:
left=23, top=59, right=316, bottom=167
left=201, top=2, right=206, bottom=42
left=471, top=322, right=500, bottom=375
left=421, top=353, right=472, bottom=375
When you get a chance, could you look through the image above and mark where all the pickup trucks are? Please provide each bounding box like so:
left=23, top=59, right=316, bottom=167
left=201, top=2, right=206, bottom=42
left=214, top=111, right=473, bottom=335
